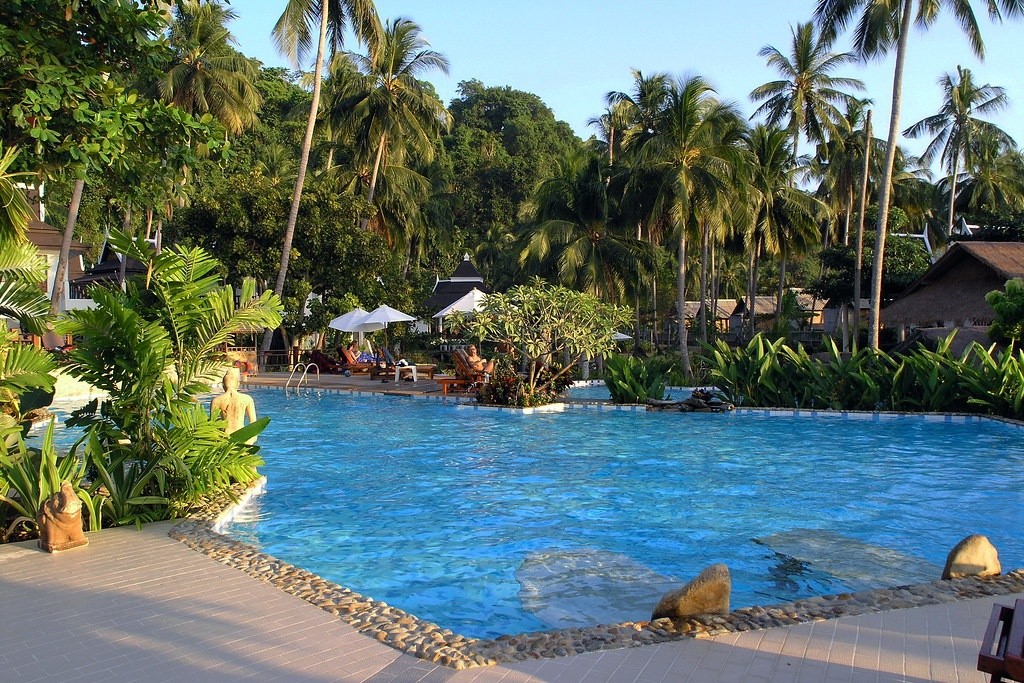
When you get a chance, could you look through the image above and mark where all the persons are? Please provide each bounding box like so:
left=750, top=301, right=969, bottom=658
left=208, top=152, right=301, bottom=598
left=346, top=340, right=378, bottom=363
left=466, top=344, right=495, bottom=375
left=210, top=368, right=258, bottom=446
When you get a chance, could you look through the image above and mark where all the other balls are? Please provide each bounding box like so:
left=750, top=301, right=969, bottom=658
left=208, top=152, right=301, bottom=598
left=344, top=370, right=351, bottom=377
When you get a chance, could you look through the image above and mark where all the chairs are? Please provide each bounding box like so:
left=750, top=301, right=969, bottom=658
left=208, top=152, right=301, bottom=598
left=437, top=348, right=517, bottom=396
left=377, top=346, right=437, bottom=379
left=340, top=347, right=385, bottom=375
left=975, top=598, right=1024, bottom=683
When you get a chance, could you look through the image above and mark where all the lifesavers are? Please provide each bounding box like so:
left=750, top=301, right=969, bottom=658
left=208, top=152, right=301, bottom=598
left=234, top=359, right=252, bottom=376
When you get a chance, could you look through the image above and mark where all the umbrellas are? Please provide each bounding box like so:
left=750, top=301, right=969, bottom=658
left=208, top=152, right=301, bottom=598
left=328, top=303, right=416, bottom=350
left=432, top=287, right=518, bottom=355
left=611, top=332, right=633, bottom=340
